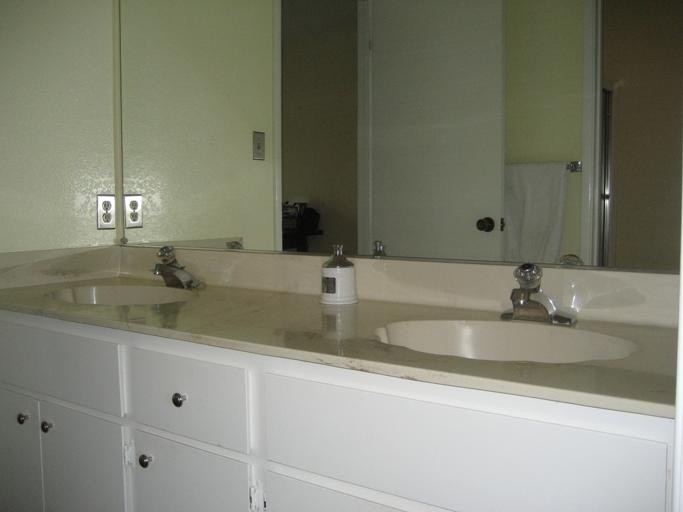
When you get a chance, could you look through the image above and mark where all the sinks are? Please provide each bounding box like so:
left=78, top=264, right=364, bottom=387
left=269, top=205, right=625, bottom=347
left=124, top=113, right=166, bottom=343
left=46, top=283, right=194, bottom=305
left=376, top=319, right=635, bottom=364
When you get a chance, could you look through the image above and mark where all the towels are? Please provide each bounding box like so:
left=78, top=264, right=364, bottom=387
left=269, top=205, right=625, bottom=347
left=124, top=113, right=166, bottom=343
left=505, top=160, right=567, bottom=267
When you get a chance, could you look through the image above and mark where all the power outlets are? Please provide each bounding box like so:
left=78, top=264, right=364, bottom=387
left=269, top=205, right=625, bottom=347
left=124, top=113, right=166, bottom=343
left=124, top=194, right=143, bottom=228
left=97, top=194, right=115, bottom=228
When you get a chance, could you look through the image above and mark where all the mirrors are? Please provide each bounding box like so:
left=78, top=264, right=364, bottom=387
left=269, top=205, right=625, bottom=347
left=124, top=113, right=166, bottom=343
left=120, top=0, right=682, bottom=274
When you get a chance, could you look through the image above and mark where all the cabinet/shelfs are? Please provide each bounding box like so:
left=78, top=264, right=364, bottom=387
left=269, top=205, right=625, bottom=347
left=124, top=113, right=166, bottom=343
left=254, top=355, right=675, bottom=512
left=127, top=331, right=253, bottom=511
left=0, top=311, right=127, bottom=512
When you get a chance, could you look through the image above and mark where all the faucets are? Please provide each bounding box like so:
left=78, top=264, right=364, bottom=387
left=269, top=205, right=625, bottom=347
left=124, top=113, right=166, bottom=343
left=559, top=253, right=583, bottom=268
left=226, top=242, right=243, bottom=250
left=501, top=263, right=578, bottom=326
left=153, top=246, right=198, bottom=289
